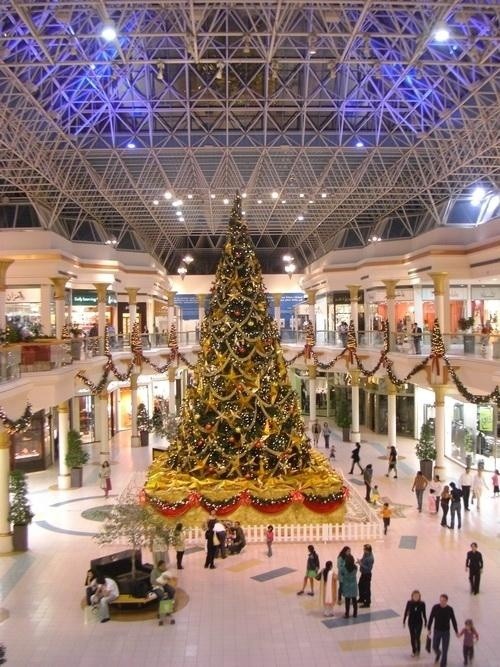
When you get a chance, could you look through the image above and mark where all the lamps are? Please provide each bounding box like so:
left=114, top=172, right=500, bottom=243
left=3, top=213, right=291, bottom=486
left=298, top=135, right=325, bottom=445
left=177, top=237, right=194, bottom=280
left=102, top=19, right=116, bottom=40
left=282, top=236, right=295, bottom=280
left=434, top=21, right=449, bottom=41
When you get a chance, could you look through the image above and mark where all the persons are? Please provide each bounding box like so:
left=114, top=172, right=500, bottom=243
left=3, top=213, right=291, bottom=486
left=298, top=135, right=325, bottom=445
left=338, top=318, right=349, bottom=348
left=107, top=323, right=117, bottom=350
left=151, top=560, right=176, bottom=609
left=152, top=571, right=177, bottom=626
left=410, top=463, right=500, bottom=530
left=321, top=421, right=331, bottom=448
left=371, top=483, right=381, bottom=508
left=311, top=418, right=322, bottom=448
left=379, top=502, right=392, bottom=534
left=428, top=593, right=460, bottom=666
left=355, top=544, right=375, bottom=609
left=297, top=544, right=319, bottom=596
left=89, top=573, right=121, bottom=624
left=81, top=568, right=97, bottom=611
left=336, top=545, right=351, bottom=605
left=464, top=542, right=483, bottom=597
left=328, top=445, right=336, bottom=461
left=411, top=323, right=423, bottom=355
left=174, top=523, right=186, bottom=569
left=402, top=589, right=427, bottom=657
left=349, top=441, right=365, bottom=475
left=361, top=464, right=373, bottom=502
left=384, top=446, right=398, bottom=479
left=267, top=525, right=274, bottom=558
left=143, top=323, right=152, bottom=348
left=98, top=460, right=113, bottom=497
left=480, top=323, right=491, bottom=345
left=152, top=323, right=160, bottom=345
left=319, top=560, right=339, bottom=618
left=341, top=553, right=358, bottom=618
left=203, top=519, right=246, bottom=569
left=457, top=618, right=481, bottom=666
left=90, top=322, right=98, bottom=335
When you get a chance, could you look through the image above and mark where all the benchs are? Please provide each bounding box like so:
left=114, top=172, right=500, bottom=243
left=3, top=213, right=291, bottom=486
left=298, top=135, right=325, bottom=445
left=100, top=576, right=178, bottom=607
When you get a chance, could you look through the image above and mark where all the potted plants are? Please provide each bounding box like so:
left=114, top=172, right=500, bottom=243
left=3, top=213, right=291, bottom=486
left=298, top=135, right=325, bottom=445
left=137, top=404, right=152, bottom=447
left=415, top=420, right=437, bottom=481
left=64, top=429, right=90, bottom=487
left=7, top=468, right=35, bottom=551
left=463, top=429, right=473, bottom=469
left=336, top=394, right=352, bottom=441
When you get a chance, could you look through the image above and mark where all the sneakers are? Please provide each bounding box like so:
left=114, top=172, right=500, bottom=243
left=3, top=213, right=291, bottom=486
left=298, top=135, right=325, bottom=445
left=101, top=618, right=110, bottom=623
left=357, top=599, right=370, bottom=608
left=297, top=590, right=313, bottom=595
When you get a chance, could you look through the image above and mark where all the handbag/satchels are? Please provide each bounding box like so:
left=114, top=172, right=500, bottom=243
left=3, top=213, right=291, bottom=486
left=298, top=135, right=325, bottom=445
left=213, top=535, right=220, bottom=546
left=426, top=635, right=430, bottom=652
left=159, top=599, right=173, bottom=614
